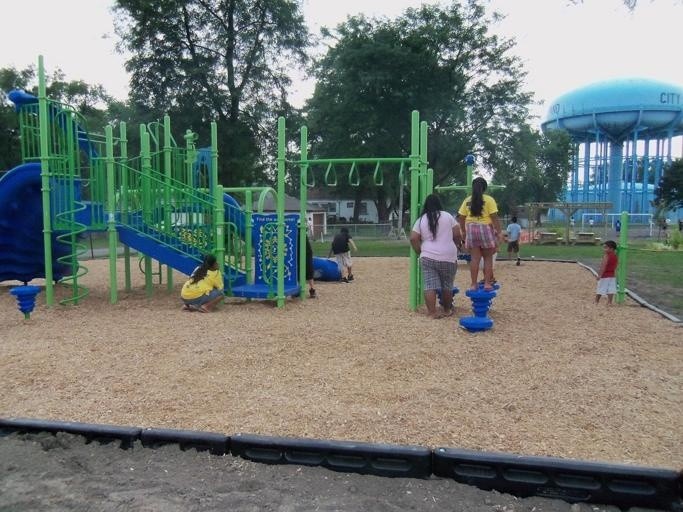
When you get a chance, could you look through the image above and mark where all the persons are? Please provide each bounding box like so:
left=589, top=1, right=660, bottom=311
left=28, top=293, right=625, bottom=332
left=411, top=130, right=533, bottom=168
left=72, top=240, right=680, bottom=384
left=506, top=217, right=522, bottom=266
left=292, top=222, right=316, bottom=298
left=588, top=217, right=594, bottom=228
left=569, top=216, right=574, bottom=228
left=179, top=254, right=225, bottom=314
left=410, top=178, right=506, bottom=320
left=327, top=226, right=357, bottom=283
left=594, top=240, right=618, bottom=305
left=616, top=220, right=621, bottom=233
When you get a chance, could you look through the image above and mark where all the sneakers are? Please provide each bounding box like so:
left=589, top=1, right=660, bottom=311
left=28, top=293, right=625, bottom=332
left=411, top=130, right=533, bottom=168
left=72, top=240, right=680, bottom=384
left=309, top=289, right=315, bottom=297
left=347, top=275, right=354, bottom=282
left=340, top=277, right=350, bottom=284
left=516, top=257, right=521, bottom=266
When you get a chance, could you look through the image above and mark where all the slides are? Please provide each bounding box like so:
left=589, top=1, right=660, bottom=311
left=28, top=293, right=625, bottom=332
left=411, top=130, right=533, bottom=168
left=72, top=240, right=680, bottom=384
left=209, top=190, right=343, bottom=282
